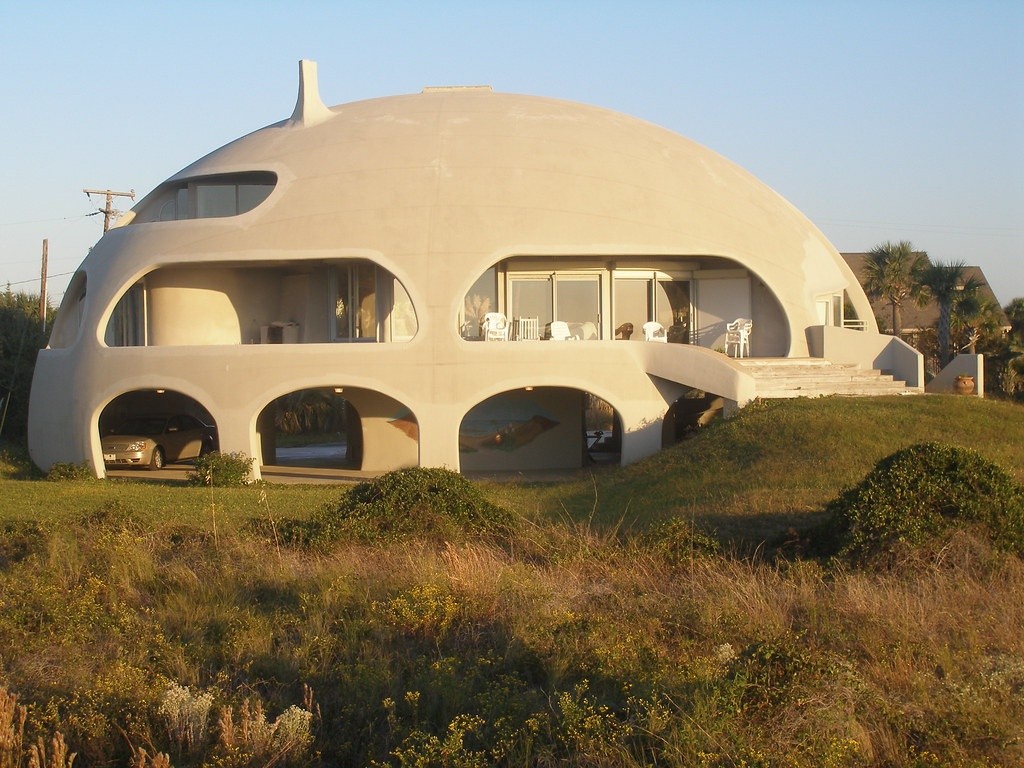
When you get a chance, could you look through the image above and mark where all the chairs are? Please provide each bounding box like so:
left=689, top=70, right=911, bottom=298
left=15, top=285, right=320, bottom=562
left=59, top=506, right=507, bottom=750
left=517, top=316, right=541, bottom=341
left=725, top=318, right=753, bottom=358
left=482, top=313, right=509, bottom=341
left=643, top=321, right=667, bottom=343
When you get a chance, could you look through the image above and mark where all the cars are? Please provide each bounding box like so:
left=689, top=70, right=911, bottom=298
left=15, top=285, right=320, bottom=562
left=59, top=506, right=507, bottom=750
left=101, top=412, right=216, bottom=471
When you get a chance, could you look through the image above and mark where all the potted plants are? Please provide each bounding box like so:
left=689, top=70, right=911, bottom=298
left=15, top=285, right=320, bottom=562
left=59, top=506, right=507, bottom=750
left=953, top=372, right=974, bottom=395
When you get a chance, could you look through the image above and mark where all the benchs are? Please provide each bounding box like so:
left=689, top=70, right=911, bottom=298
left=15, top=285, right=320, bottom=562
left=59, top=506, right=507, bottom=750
left=549, top=321, right=598, bottom=340
